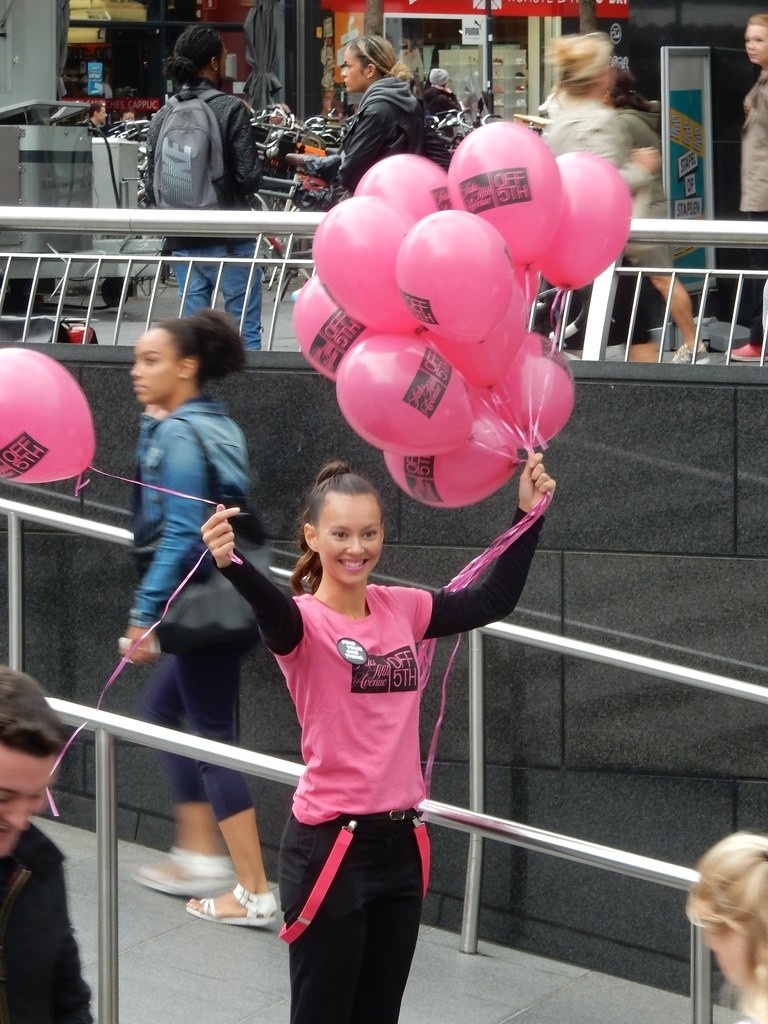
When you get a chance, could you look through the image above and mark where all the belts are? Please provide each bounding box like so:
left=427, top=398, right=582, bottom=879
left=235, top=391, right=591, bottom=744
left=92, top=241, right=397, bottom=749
left=326, top=808, right=416, bottom=827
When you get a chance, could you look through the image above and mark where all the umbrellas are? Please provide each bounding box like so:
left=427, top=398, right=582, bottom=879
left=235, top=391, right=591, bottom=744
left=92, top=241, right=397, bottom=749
left=242, top=0, right=281, bottom=112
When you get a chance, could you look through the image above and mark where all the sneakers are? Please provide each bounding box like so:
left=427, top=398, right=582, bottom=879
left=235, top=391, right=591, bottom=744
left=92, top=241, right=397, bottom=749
left=725, top=342, right=768, bottom=362
left=672, top=343, right=710, bottom=365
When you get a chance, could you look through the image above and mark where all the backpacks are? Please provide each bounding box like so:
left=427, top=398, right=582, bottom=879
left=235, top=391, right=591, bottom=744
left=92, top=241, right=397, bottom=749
left=152, top=89, right=228, bottom=210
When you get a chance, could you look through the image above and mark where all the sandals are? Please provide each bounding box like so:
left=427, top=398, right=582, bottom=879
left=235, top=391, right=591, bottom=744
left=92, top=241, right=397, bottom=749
left=185, top=883, right=278, bottom=927
left=132, top=846, right=236, bottom=898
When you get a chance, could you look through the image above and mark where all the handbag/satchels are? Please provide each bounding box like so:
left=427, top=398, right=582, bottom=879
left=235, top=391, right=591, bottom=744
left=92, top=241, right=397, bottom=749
left=125, top=415, right=272, bottom=660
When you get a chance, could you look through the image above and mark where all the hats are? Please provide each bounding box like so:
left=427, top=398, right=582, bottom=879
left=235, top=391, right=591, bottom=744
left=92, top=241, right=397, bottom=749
left=429, top=68, right=449, bottom=87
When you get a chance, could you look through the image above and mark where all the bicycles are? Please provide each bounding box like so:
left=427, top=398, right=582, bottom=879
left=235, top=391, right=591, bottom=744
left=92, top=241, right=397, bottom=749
left=116, top=103, right=540, bottom=301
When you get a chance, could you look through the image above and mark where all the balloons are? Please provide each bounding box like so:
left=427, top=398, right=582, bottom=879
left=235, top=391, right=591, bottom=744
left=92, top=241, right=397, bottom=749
left=292, top=114, right=632, bottom=509
left=0, top=348, right=94, bottom=483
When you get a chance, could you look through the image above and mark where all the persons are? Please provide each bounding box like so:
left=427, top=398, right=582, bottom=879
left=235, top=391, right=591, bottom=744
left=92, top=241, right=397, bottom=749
left=338, top=34, right=461, bottom=209
left=145, top=25, right=265, bottom=353
left=0, top=664, right=94, bottom=1024
left=533, top=31, right=709, bottom=365
left=73, top=104, right=136, bottom=138
left=726, top=13, right=768, bottom=361
left=201, top=452, right=558, bottom=1024
left=685, top=828, right=768, bottom=1024
left=121, top=308, right=278, bottom=926
